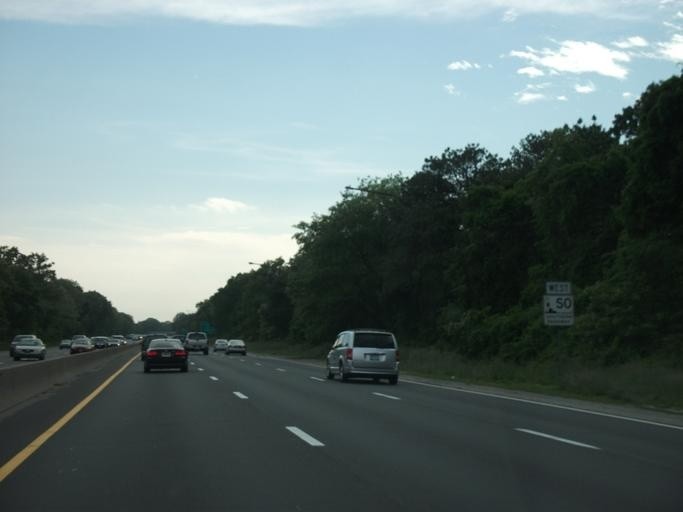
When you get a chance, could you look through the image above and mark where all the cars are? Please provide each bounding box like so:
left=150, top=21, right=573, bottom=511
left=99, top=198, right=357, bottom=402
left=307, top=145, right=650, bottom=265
left=59, top=331, right=210, bottom=372
left=326, top=329, right=401, bottom=385
left=214, top=338, right=247, bottom=356
left=9, top=334, right=48, bottom=360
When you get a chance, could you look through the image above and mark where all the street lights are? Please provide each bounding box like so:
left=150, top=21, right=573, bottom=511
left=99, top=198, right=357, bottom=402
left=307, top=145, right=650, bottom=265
left=346, top=185, right=401, bottom=200
left=249, top=261, right=262, bottom=266
